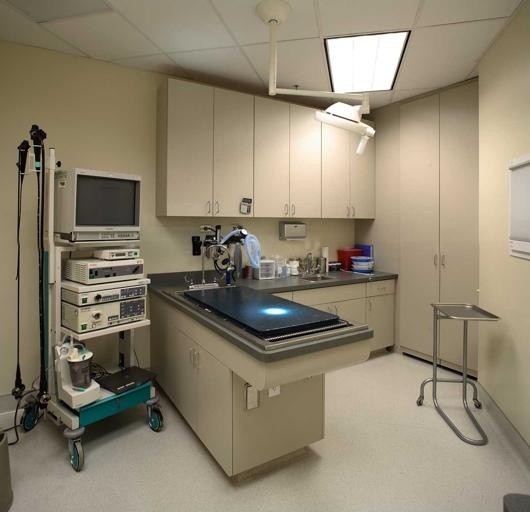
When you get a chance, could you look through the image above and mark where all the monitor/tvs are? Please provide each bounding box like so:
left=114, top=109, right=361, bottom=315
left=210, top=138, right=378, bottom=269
left=44, top=168, right=142, bottom=242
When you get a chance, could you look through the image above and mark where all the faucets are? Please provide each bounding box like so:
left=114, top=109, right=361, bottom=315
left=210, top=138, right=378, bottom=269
left=202, top=245, right=231, bottom=280
left=306, top=253, right=312, bottom=271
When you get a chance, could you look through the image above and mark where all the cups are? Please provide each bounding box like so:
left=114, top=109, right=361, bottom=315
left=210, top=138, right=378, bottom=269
left=288, top=260, right=300, bottom=275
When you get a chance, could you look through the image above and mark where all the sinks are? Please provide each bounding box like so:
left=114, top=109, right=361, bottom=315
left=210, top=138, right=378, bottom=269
left=300, top=276, right=333, bottom=281
left=189, top=282, right=236, bottom=290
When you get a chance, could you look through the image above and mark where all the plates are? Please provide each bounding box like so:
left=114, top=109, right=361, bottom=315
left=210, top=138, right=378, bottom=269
left=350, top=256, right=375, bottom=273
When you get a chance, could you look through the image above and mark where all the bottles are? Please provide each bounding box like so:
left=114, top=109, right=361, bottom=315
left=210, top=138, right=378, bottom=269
left=316, top=257, right=326, bottom=274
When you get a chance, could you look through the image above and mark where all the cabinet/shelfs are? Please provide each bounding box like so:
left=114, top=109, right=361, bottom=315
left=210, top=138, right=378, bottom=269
left=398, top=80, right=479, bottom=381
left=271, top=291, right=292, bottom=302
left=148, top=290, right=327, bottom=484
left=364, top=278, right=395, bottom=351
left=155, top=78, right=376, bottom=220
left=292, top=282, right=367, bottom=326
